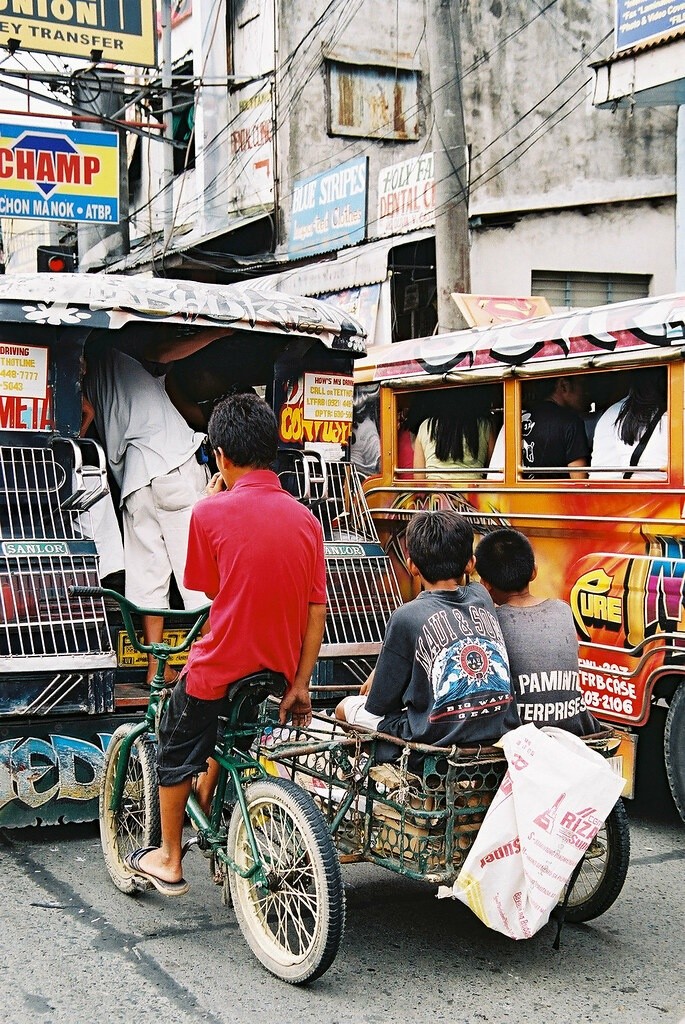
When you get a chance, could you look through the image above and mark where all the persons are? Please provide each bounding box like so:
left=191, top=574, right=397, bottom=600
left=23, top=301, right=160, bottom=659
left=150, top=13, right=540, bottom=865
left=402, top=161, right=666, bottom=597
left=487, top=375, right=592, bottom=478
left=410, top=386, right=495, bottom=479
left=83, top=327, right=237, bottom=690
left=475, top=530, right=601, bottom=737
left=124, top=394, right=327, bottom=896
left=588, top=366, right=668, bottom=481
left=335, top=510, right=523, bottom=764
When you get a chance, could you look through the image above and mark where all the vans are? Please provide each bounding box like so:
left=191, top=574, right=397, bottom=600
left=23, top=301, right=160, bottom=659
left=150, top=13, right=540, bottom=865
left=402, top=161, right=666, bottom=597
left=351, top=291, right=685, bottom=825
left=0, top=274, right=418, bottom=830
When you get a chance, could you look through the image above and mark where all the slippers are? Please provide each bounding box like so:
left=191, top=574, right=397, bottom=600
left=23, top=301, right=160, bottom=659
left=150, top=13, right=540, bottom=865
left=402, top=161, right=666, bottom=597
left=187, top=778, right=207, bottom=831
left=147, top=670, right=183, bottom=688
left=124, top=846, right=189, bottom=896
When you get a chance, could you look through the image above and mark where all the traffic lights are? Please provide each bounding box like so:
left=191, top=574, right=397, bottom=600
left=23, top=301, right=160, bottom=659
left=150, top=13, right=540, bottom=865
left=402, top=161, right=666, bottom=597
left=37, top=246, right=76, bottom=273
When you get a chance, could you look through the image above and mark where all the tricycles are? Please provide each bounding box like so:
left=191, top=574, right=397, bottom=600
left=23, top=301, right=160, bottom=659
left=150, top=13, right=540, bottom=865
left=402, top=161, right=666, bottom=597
left=68, top=585, right=635, bottom=987
